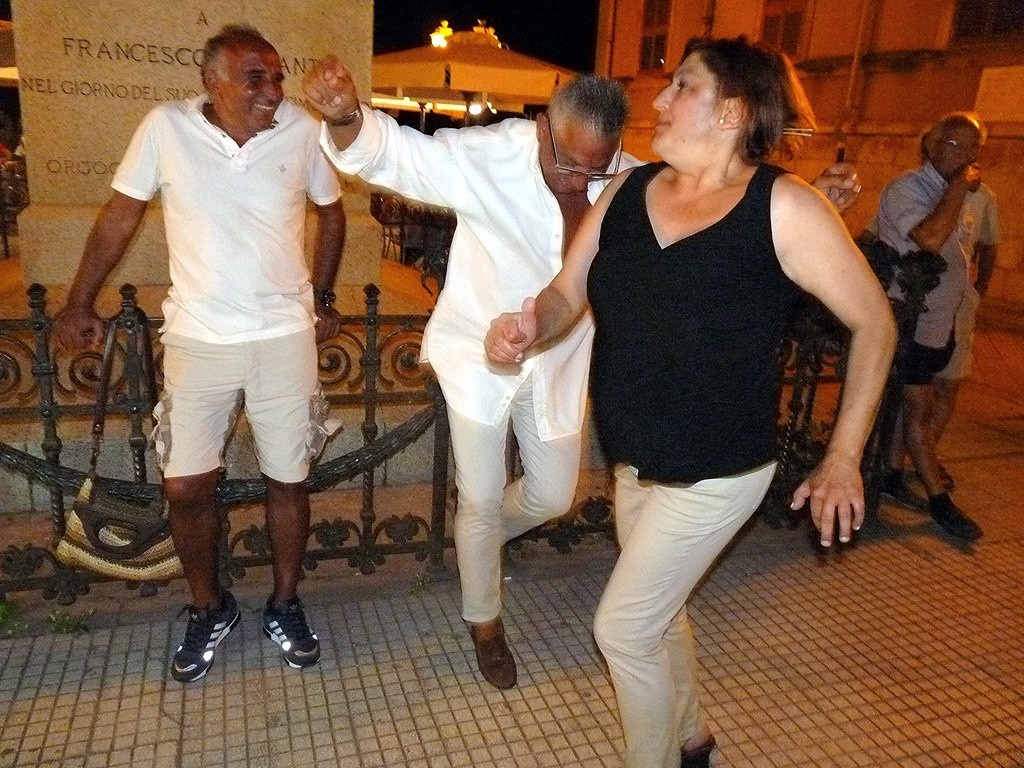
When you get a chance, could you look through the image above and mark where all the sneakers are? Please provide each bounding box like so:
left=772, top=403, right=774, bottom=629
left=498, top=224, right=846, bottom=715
left=262, top=591, right=320, bottom=669
left=171, top=590, right=242, bottom=682
left=470, top=618, right=517, bottom=690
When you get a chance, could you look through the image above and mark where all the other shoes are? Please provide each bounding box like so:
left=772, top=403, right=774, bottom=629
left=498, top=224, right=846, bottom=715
left=928, top=492, right=983, bottom=539
left=880, top=469, right=926, bottom=509
left=937, top=466, right=955, bottom=490
left=681, top=733, right=716, bottom=768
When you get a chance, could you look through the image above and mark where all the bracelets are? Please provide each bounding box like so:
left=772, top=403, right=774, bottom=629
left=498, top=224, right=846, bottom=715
left=321, top=103, right=363, bottom=128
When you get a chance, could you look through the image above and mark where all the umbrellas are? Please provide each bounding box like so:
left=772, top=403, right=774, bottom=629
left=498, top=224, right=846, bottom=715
left=371, top=18, right=582, bottom=137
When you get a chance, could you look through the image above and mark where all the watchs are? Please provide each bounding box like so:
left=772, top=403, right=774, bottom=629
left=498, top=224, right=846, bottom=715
left=313, top=286, right=338, bottom=308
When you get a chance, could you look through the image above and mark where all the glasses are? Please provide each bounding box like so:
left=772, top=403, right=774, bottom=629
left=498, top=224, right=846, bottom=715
left=543, top=111, right=623, bottom=180
left=940, top=138, right=981, bottom=154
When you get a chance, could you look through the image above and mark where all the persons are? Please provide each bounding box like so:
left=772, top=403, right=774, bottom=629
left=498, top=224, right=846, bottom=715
left=483, top=30, right=899, bottom=767
left=298, top=69, right=861, bottom=691
left=50, top=21, right=347, bottom=684
left=0, top=134, right=26, bottom=259
left=858, top=110, right=1001, bottom=543
